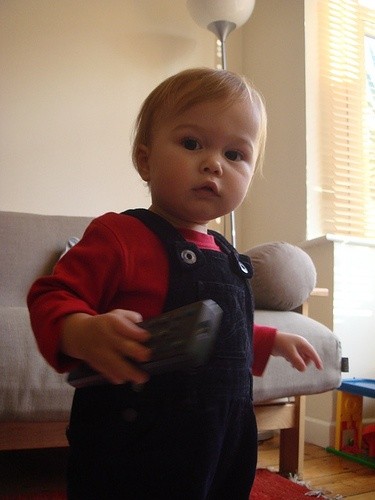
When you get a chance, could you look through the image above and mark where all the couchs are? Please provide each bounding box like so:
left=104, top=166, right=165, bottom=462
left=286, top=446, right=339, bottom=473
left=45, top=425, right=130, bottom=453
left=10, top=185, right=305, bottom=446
left=0, top=211, right=350, bottom=483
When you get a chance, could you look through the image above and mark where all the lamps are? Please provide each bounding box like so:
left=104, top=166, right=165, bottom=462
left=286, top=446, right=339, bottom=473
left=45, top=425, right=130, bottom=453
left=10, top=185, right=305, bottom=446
left=188, top=0, right=256, bottom=250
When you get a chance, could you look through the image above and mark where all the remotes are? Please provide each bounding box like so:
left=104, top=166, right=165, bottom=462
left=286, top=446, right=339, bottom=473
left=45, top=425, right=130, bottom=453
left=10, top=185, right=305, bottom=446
left=68, top=301, right=223, bottom=390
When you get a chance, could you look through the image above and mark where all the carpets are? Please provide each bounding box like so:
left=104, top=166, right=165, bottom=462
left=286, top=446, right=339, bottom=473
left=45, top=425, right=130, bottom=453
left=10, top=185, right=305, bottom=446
left=0, top=468, right=329, bottom=500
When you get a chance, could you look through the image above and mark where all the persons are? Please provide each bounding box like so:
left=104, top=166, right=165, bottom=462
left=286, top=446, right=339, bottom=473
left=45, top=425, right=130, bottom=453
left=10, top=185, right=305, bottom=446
left=28, top=68, right=324, bottom=500
